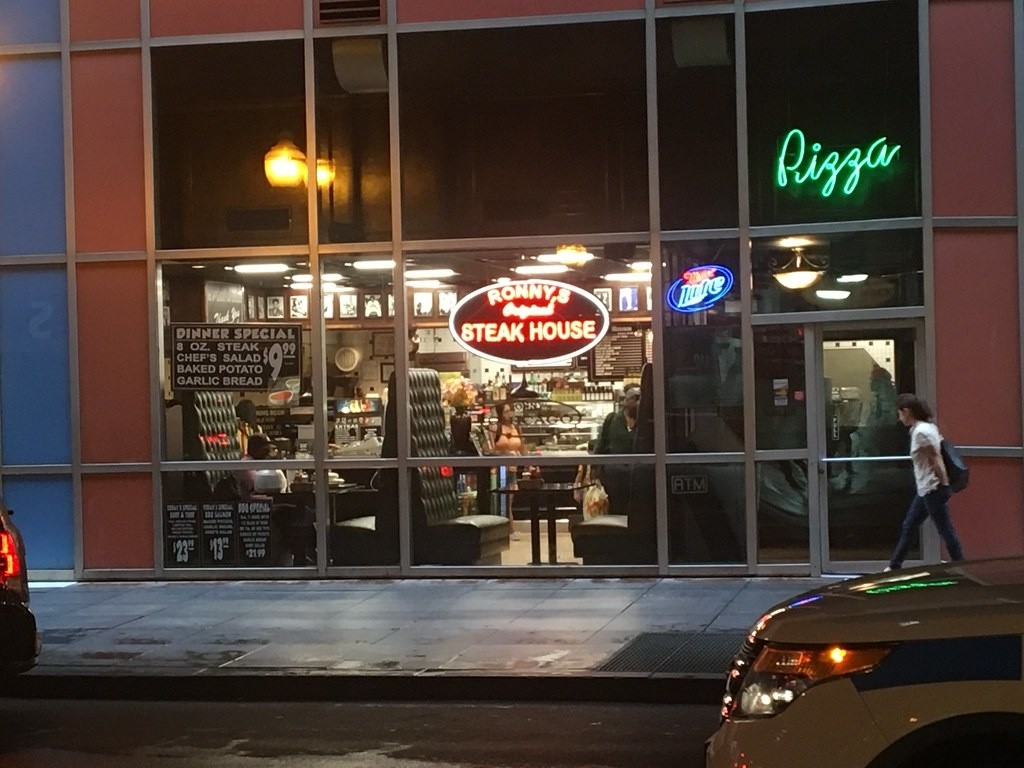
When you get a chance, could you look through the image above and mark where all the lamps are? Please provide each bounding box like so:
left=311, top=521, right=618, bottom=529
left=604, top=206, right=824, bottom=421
left=510, top=369, right=540, bottom=398
left=775, top=246, right=827, bottom=289
left=265, top=139, right=306, bottom=187
left=304, top=159, right=336, bottom=188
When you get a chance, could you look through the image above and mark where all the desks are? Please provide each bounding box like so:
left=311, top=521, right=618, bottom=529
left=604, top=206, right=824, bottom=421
left=489, top=483, right=594, bottom=565
left=266, top=484, right=365, bottom=571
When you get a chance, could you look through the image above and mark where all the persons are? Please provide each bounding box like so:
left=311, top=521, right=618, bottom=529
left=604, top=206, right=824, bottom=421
left=884, top=393, right=967, bottom=572
left=235, top=400, right=263, bottom=455
left=236, top=435, right=292, bottom=496
left=848, top=368, right=898, bottom=454
left=575, top=384, right=639, bottom=511
left=489, top=403, right=527, bottom=542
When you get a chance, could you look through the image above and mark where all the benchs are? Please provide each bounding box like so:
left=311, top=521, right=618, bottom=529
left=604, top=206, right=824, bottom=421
left=326, top=368, right=513, bottom=566
left=166, top=392, right=291, bottom=568
left=575, top=362, right=659, bottom=564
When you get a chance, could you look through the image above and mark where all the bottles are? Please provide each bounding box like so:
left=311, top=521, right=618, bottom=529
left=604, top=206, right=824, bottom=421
left=529, top=369, right=548, bottom=396
left=485, top=372, right=508, bottom=401
left=551, top=386, right=614, bottom=401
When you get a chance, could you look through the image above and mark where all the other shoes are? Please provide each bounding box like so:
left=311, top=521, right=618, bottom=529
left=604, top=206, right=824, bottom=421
left=510, top=530, right=520, bottom=540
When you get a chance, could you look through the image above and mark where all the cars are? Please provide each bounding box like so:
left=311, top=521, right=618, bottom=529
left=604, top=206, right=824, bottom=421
left=832, top=386, right=864, bottom=403
left=699, top=556, right=1024, bottom=768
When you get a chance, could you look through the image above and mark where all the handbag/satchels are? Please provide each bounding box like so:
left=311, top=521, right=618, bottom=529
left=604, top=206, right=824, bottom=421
left=939, top=439, right=970, bottom=492
left=582, top=484, right=610, bottom=521
